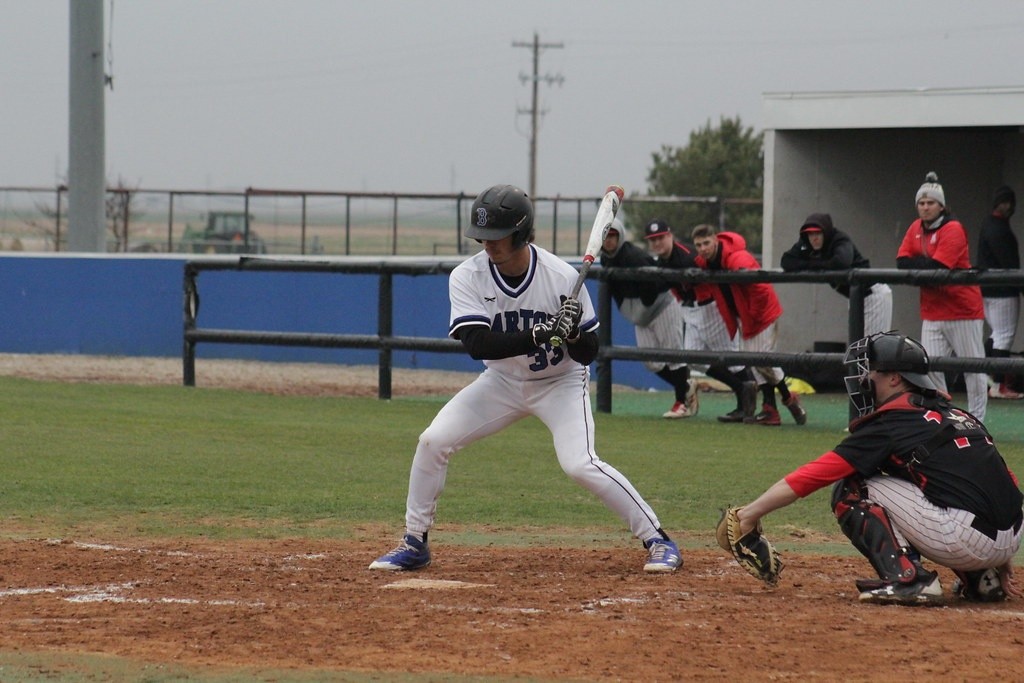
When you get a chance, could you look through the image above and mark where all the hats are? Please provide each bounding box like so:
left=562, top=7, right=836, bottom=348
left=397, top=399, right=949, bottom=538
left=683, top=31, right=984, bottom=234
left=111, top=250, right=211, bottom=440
left=915, top=171, right=945, bottom=208
left=643, top=220, right=671, bottom=239
left=991, top=186, right=1015, bottom=208
left=801, top=225, right=823, bottom=232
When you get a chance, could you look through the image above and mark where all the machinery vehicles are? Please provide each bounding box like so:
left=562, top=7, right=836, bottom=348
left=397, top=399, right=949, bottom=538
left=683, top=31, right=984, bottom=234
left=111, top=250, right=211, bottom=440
left=176, top=208, right=267, bottom=255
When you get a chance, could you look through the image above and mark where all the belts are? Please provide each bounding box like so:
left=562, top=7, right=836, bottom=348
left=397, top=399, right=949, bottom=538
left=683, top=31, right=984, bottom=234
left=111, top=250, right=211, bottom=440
left=863, top=289, right=873, bottom=298
left=682, top=299, right=712, bottom=306
left=971, top=516, right=1022, bottom=541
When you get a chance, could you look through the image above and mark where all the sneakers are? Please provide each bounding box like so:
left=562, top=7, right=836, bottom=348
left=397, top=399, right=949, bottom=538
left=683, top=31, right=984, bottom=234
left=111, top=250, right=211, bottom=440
left=717, top=409, right=746, bottom=422
left=661, top=400, right=693, bottom=418
left=784, top=397, right=806, bottom=425
left=743, top=404, right=780, bottom=425
left=856, top=568, right=947, bottom=607
left=740, top=380, right=756, bottom=414
left=642, top=539, right=683, bottom=573
left=988, top=383, right=1024, bottom=399
left=952, top=568, right=1005, bottom=601
left=685, top=378, right=699, bottom=416
left=368, top=532, right=431, bottom=570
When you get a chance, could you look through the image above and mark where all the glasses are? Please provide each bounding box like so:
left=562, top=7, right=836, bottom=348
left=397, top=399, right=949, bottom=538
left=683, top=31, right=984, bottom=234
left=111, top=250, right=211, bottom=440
left=606, top=234, right=618, bottom=238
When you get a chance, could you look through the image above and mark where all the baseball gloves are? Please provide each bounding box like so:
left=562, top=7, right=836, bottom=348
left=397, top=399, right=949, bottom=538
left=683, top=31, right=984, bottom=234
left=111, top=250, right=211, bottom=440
left=715, top=502, right=789, bottom=590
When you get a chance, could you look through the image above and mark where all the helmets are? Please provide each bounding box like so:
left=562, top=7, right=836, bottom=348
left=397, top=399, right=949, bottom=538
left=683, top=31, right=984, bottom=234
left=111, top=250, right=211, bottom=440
left=463, top=185, right=533, bottom=245
left=869, top=334, right=937, bottom=390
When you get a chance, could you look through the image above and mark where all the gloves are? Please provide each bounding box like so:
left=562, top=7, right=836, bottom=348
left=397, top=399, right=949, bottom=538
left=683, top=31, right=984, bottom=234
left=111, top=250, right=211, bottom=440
left=533, top=314, right=574, bottom=339
left=557, top=295, right=583, bottom=325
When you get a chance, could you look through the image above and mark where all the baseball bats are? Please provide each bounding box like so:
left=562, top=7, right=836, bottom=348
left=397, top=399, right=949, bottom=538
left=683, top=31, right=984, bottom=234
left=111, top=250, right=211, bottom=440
left=550, top=183, right=625, bottom=348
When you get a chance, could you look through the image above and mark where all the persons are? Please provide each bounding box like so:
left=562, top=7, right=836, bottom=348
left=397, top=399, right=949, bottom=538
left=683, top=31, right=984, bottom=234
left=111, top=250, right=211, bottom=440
left=976, top=186, right=1024, bottom=399
left=368, top=184, right=684, bottom=574
left=600, top=219, right=698, bottom=417
left=691, top=225, right=806, bottom=426
left=716, top=333, right=1023, bottom=602
left=781, top=212, right=893, bottom=336
left=896, top=172, right=988, bottom=424
left=641, top=219, right=757, bottom=422
